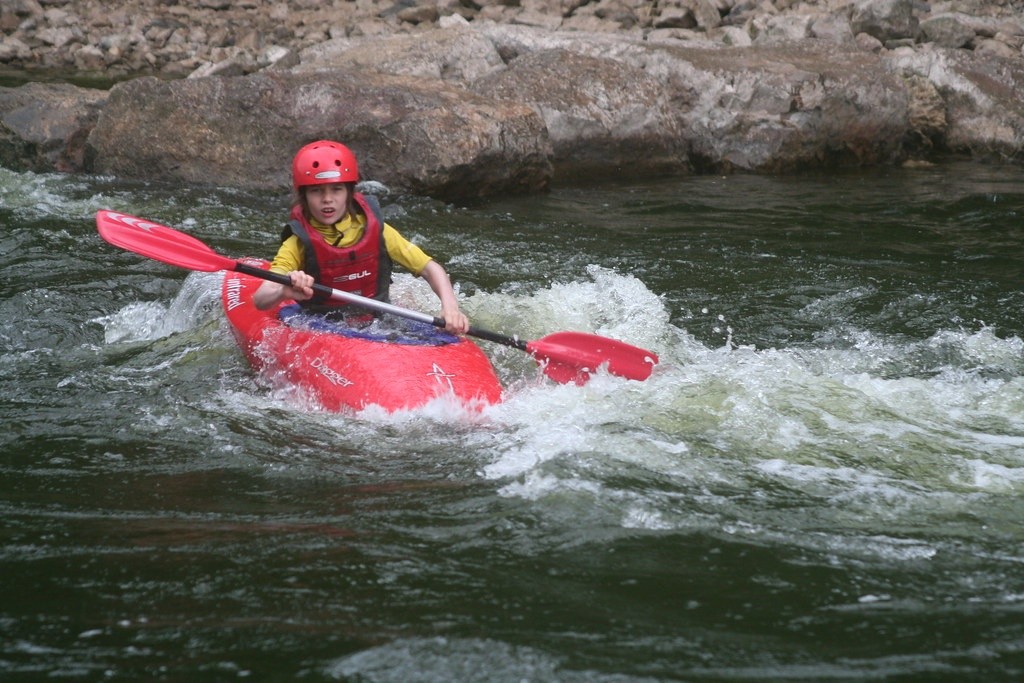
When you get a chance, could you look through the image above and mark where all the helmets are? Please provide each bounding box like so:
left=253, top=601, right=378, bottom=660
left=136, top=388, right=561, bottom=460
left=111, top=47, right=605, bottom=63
left=292, top=140, right=360, bottom=191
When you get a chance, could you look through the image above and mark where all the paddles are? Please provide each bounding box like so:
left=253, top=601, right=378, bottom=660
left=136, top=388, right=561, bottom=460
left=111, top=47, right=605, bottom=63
left=92, top=207, right=661, bottom=391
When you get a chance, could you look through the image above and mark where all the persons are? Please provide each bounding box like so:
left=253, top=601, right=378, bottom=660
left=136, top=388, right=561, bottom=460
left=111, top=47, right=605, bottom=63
left=253, top=140, right=471, bottom=338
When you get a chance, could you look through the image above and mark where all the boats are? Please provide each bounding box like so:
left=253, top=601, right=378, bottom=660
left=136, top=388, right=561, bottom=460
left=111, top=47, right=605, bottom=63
left=222, top=257, right=505, bottom=419
